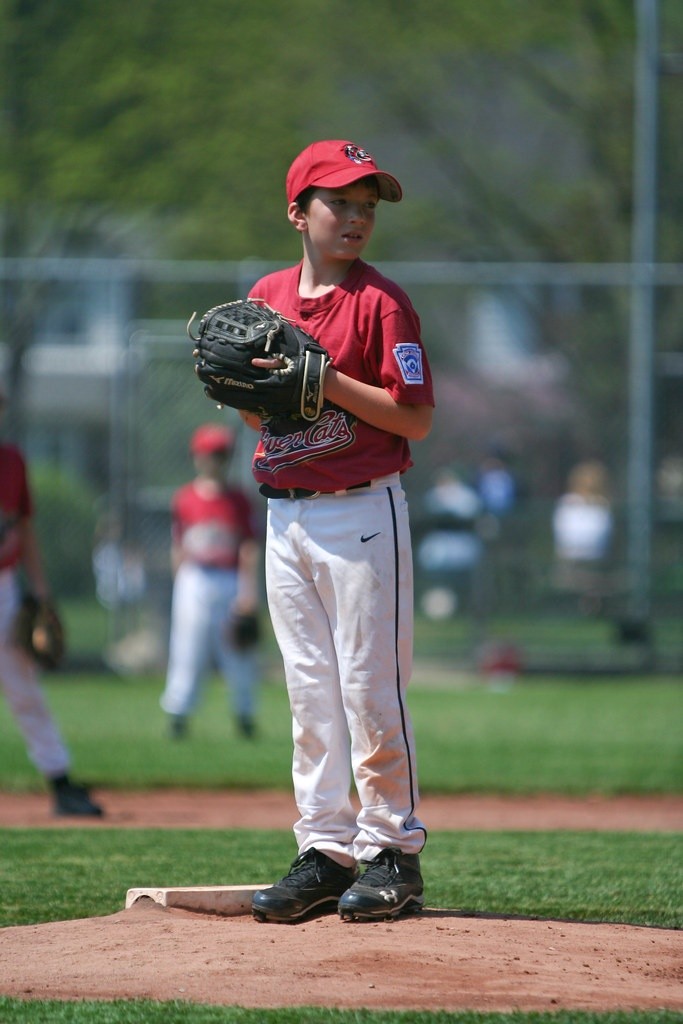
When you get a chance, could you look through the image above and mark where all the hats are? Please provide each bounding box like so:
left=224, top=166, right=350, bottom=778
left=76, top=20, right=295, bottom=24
left=285, top=139, right=403, bottom=205
left=190, top=426, right=236, bottom=452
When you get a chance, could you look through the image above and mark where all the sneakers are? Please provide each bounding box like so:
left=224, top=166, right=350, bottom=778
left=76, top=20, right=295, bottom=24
left=337, top=845, right=424, bottom=923
left=250, top=847, right=362, bottom=921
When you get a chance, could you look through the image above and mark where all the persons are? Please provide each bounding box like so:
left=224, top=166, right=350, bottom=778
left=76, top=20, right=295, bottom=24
left=159, top=426, right=262, bottom=739
left=192, top=142, right=433, bottom=922
left=0, top=440, right=101, bottom=816
left=550, top=451, right=617, bottom=567
left=417, top=446, right=515, bottom=581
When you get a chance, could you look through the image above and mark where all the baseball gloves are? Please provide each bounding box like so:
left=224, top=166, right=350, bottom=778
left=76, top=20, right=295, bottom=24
left=183, top=295, right=333, bottom=433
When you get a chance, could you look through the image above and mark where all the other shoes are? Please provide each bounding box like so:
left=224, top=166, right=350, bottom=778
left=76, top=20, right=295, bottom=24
left=53, top=774, right=104, bottom=818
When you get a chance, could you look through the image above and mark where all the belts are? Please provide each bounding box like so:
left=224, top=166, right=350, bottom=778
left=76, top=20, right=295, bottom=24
left=259, top=480, right=371, bottom=499
